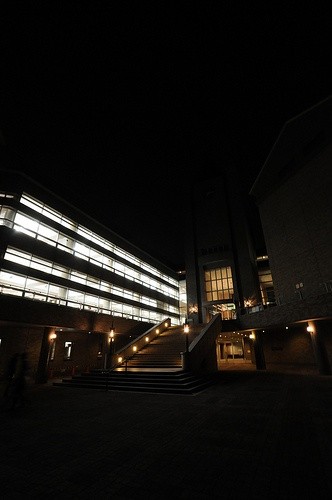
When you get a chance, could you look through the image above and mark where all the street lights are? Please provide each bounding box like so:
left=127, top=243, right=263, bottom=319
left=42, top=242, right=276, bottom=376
left=183, top=318, right=190, bottom=352
left=109, top=320, right=114, bottom=355
